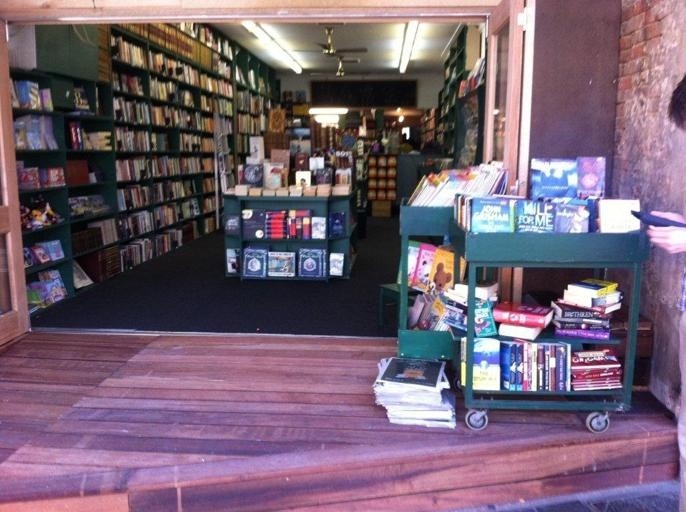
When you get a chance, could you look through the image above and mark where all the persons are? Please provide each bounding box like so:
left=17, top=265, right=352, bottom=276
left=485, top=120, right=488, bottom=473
left=646, top=72, right=686, bottom=512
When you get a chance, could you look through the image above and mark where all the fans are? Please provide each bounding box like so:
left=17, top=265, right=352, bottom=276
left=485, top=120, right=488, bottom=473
left=291, top=25, right=370, bottom=79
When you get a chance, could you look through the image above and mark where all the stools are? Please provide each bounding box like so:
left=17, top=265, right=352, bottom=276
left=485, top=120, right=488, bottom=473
left=377, top=282, right=422, bottom=327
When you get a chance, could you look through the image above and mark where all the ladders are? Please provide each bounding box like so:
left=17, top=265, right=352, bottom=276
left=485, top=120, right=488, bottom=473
left=213, top=112, right=236, bottom=227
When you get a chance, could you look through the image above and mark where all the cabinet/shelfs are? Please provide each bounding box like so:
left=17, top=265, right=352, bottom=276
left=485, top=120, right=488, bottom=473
left=35, top=17, right=281, bottom=274
left=394, top=197, right=454, bottom=361
left=9, top=69, right=115, bottom=317
left=418, top=25, right=485, bottom=169
left=448, top=219, right=649, bottom=434
left=223, top=183, right=358, bottom=282
